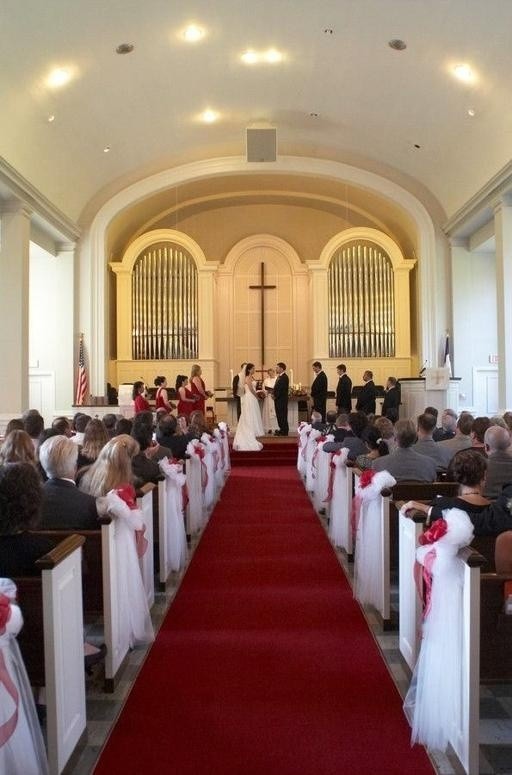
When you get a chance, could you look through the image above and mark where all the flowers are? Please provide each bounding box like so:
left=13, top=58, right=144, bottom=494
left=168, top=401, right=176, bottom=408
left=256, top=390, right=267, bottom=401
left=206, top=390, right=215, bottom=398
left=192, top=393, right=201, bottom=400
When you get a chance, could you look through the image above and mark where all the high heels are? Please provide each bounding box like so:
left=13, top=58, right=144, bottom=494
left=85, top=643, right=107, bottom=676
left=36, top=704, right=46, bottom=726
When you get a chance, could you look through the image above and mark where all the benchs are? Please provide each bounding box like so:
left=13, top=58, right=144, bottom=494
left=2, top=425, right=229, bottom=775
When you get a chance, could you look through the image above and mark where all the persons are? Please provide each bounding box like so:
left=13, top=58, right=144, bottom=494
left=308, top=361, right=402, bottom=428
left=232, top=361, right=290, bottom=451
left=131, top=363, right=215, bottom=424
left=305, top=406, right=512, bottom=592
left=1, top=407, right=215, bottom=726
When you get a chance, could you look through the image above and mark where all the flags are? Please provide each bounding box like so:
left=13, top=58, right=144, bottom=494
left=72, top=339, right=93, bottom=406
left=442, top=336, right=455, bottom=380
